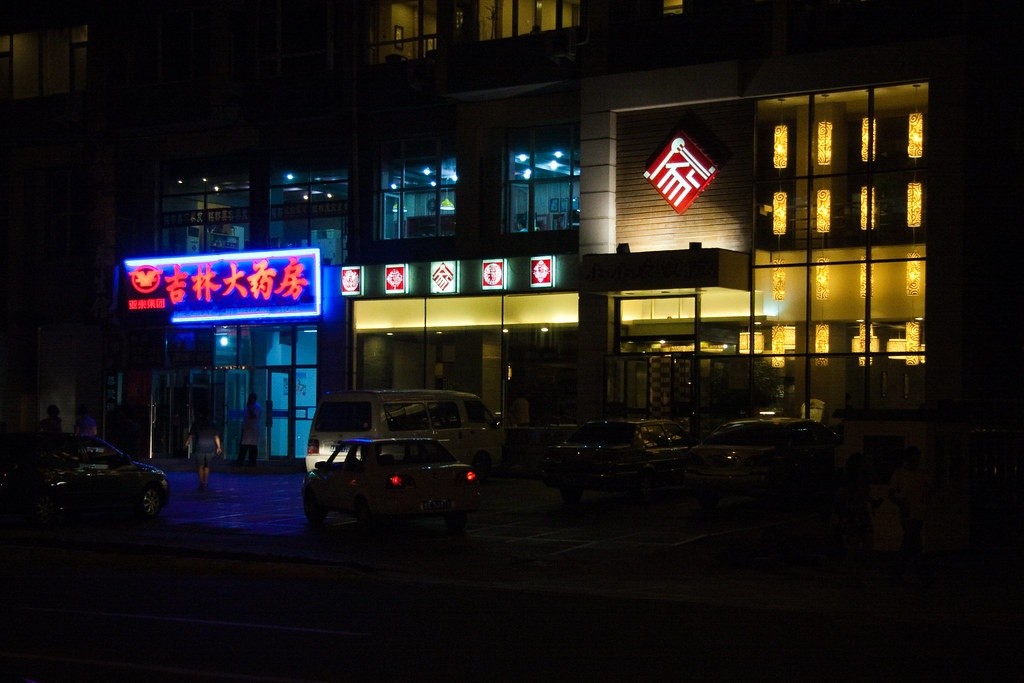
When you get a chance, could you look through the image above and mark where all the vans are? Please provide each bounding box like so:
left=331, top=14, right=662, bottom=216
left=304, top=387, right=508, bottom=477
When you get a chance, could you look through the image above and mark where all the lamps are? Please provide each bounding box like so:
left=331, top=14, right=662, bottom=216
left=440, top=178, right=456, bottom=210
left=392, top=197, right=408, bottom=212
left=737, top=80, right=927, bottom=368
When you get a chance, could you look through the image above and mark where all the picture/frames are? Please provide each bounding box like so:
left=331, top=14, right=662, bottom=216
left=394, top=25, right=404, bottom=51
left=572, top=197, right=579, bottom=210
left=559, top=198, right=569, bottom=212
left=550, top=198, right=558, bottom=212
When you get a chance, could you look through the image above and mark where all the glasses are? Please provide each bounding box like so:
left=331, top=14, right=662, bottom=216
left=249, top=400, right=256, bottom=402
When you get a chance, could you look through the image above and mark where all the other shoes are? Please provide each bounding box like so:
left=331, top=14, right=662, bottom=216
left=246, top=464, right=257, bottom=467
left=199, top=482, right=208, bottom=487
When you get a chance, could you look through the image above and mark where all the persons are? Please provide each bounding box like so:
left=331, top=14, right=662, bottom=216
left=832, top=453, right=874, bottom=579
left=185, top=407, right=223, bottom=489
left=74, top=407, right=97, bottom=436
left=887, top=447, right=933, bottom=577
left=236, top=393, right=261, bottom=464
left=511, top=390, right=530, bottom=426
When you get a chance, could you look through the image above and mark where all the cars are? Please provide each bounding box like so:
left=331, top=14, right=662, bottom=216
left=539, top=415, right=700, bottom=498
left=0, top=428, right=169, bottom=526
left=682, top=416, right=844, bottom=505
left=301, top=435, right=477, bottom=530
